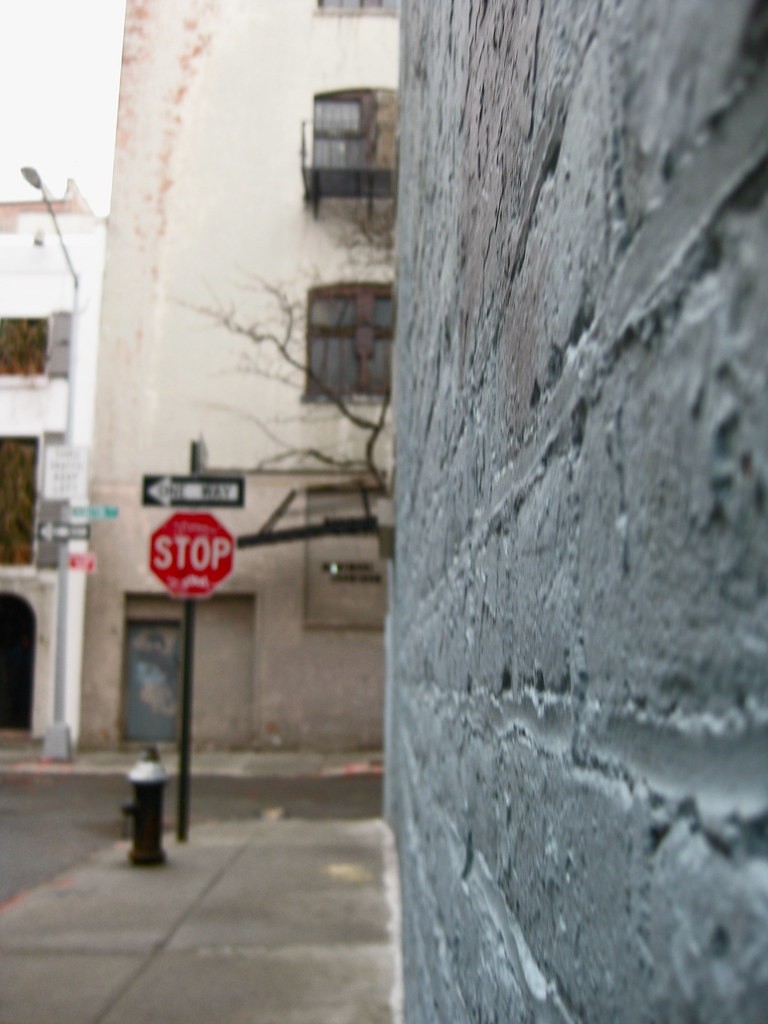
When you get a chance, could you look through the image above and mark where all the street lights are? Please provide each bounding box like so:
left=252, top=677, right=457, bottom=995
left=22, top=164, right=82, bottom=764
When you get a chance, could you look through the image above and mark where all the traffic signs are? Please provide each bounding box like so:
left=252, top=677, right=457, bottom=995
left=140, top=472, right=247, bottom=511
left=34, top=521, right=88, bottom=541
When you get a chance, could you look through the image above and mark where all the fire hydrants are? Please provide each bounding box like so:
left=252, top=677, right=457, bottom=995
left=123, top=741, right=168, bottom=866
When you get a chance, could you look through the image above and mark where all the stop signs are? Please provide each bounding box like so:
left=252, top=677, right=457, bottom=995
left=149, top=511, right=236, bottom=599
